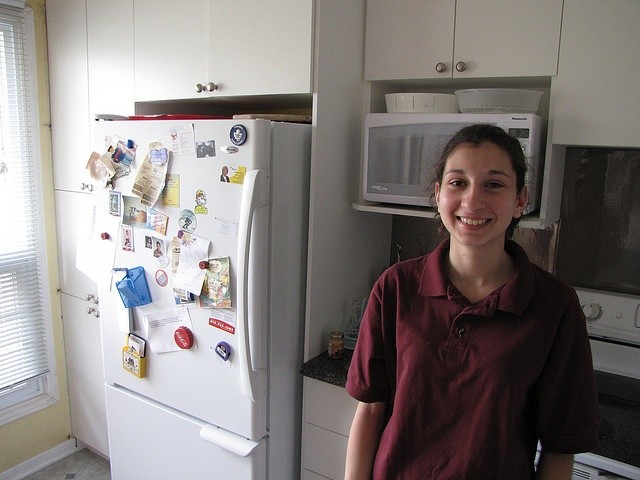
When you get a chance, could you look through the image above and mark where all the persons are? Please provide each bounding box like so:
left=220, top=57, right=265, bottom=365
left=345, top=124, right=601, bottom=480
left=153, top=241, right=163, bottom=257
left=220, top=166, right=229, bottom=182
left=146, top=238, right=151, bottom=248
left=107, top=146, right=132, bottom=166
left=124, top=230, right=131, bottom=247
left=111, top=195, right=117, bottom=211
left=182, top=218, right=192, bottom=229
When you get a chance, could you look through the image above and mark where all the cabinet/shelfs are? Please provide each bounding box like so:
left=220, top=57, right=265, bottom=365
left=45, top=1, right=134, bottom=462
left=301, top=379, right=362, bottom=479
left=553, top=1, right=640, bottom=149
left=134, top=0, right=315, bottom=101
left=363, top=0, right=567, bottom=231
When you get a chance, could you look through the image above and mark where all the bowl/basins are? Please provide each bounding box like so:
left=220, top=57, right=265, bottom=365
left=454, top=88, right=544, bottom=114
left=385, top=93, right=458, bottom=113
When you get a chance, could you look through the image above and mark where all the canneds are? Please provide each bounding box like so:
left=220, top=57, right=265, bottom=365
left=328, top=330, right=345, bottom=358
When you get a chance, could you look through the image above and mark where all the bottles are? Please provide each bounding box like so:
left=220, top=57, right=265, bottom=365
left=345, top=300, right=362, bottom=350
left=327, top=331, right=344, bottom=361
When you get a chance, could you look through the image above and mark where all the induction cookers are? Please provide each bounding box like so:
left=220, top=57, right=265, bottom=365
left=537, top=286, right=640, bottom=479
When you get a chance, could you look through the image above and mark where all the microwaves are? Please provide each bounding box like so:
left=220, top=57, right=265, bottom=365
left=364, top=113, right=544, bottom=215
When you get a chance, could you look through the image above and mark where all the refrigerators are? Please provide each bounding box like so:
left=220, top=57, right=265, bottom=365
left=94, top=120, right=311, bottom=480
